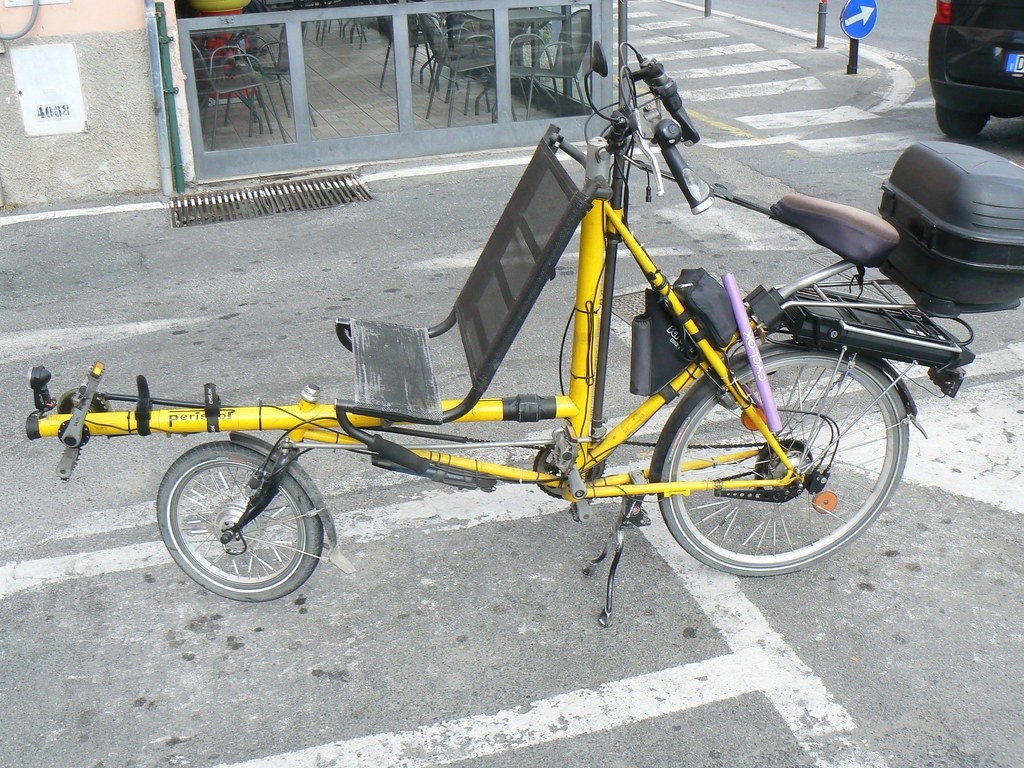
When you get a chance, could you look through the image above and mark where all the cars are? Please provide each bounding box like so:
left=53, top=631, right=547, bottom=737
left=928, top=0, right=1024, bottom=136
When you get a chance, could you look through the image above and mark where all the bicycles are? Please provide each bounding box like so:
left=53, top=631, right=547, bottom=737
left=25, top=0, right=1024, bottom=627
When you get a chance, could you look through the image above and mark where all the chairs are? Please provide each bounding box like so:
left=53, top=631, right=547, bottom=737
left=187, top=0, right=615, bottom=145
left=335, top=124, right=599, bottom=497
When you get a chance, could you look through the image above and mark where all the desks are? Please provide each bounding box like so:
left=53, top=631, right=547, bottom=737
left=465, top=7, right=567, bottom=121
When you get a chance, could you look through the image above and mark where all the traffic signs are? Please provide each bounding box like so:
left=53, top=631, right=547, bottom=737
left=838, top=0, right=877, bottom=38
